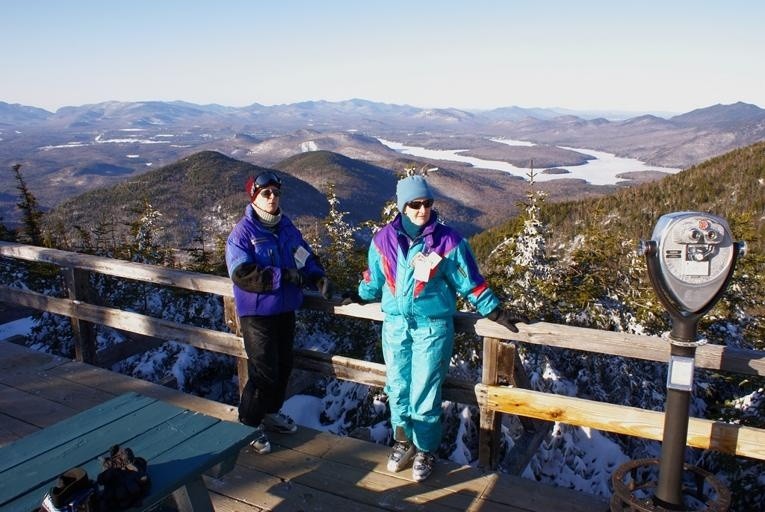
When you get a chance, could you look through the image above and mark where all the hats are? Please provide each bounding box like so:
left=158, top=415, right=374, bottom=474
left=396, top=175, right=433, bottom=214
left=246, top=175, right=281, bottom=203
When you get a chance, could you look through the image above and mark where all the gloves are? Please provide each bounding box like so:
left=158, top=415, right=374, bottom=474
left=315, top=275, right=332, bottom=301
left=488, top=307, right=530, bottom=333
left=283, top=268, right=306, bottom=289
left=339, top=287, right=368, bottom=305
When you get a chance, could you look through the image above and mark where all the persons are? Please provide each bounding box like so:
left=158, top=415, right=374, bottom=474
left=223, top=171, right=333, bottom=456
left=339, top=173, right=531, bottom=482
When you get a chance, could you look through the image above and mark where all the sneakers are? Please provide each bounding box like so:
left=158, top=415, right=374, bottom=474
left=409, top=450, right=441, bottom=482
left=249, top=423, right=272, bottom=455
left=387, top=440, right=416, bottom=473
left=262, top=409, right=297, bottom=434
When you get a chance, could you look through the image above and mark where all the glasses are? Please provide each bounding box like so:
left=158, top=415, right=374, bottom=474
left=406, top=199, right=433, bottom=209
left=260, top=189, right=282, bottom=198
left=250, top=172, right=281, bottom=197
